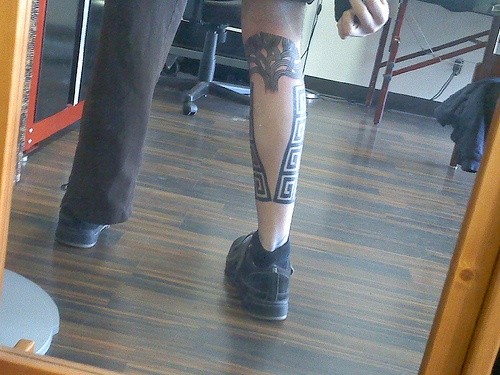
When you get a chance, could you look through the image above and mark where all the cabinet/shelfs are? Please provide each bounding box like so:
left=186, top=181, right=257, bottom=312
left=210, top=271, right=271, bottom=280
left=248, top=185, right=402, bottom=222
left=22, top=1, right=106, bottom=155
left=168, top=15, right=250, bottom=70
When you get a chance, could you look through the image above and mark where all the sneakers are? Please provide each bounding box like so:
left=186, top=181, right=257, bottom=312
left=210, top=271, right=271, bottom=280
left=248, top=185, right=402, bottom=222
left=54, top=210, right=108, bottom=246
left=226, top=229, right=293, bottom=321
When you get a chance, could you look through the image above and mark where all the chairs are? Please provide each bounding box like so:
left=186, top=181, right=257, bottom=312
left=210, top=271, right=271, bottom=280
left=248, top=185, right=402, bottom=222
left=182, top=0, right=251, bottom=116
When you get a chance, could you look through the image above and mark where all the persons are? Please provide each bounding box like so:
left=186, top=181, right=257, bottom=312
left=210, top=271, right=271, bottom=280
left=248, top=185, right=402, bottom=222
left=56, top=1, right=390, bottom=321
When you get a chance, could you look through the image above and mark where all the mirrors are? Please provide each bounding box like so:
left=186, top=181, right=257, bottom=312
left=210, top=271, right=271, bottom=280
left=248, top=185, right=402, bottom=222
left=0, top=0, right=500, bottom=375
